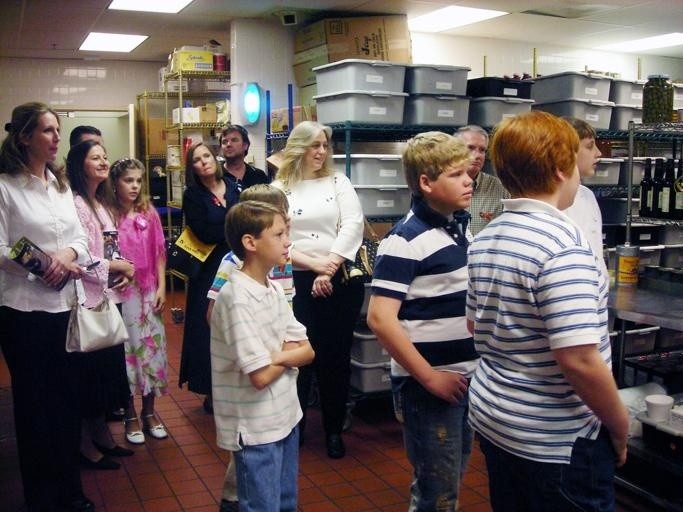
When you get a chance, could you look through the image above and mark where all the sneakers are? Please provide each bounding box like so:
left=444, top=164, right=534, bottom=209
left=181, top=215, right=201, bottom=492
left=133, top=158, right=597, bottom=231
left=222, top=497, right=240, bottom=512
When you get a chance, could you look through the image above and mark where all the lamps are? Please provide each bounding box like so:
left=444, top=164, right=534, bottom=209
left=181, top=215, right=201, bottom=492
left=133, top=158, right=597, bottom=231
left=243, top=82, right=266, bottom=124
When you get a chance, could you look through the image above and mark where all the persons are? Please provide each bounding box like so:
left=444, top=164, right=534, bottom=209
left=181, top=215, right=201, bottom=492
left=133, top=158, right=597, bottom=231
left=466, top=111, right=630, bottom=512
left=367, top=130, right=474, bottom=512
left=0, top=102, right=91, bottom=511
left=454, top=125, right=510, bottom=233
left=563, top=119, right=607, bottom=288
left=65, top=136, right=137, bottom=473
left=180, top=144, right=242, bottom=409
left=218, top=127, right=271, bottom=188
left=69, top=125, right=103, bottom=147
left=270, top=120, right=364, bottom=459
left=207, top=184, right=295, bottom=512
left=109, top=158, right=170, bottom=447
left=209, top=200, right=317, bottom=512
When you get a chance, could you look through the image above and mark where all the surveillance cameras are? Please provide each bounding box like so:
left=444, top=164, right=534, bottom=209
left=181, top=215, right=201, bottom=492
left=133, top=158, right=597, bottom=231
left=280, top=10, right=297, bottom=26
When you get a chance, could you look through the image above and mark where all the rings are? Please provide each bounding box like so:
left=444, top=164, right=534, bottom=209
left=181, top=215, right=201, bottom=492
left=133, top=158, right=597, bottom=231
left=59, top=270, right=64, bottom=277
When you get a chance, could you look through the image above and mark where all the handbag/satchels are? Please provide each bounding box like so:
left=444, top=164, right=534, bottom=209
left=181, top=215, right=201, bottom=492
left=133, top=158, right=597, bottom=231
left=66, top=243, right=129, bottom=354
left=170, top=190, right=213, bottom=279
left=334, top=172, right=383, bottom=287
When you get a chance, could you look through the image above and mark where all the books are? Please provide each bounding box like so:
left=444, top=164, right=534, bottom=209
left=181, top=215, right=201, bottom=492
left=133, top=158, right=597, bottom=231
left=10, top=237, right=67, bottom=291
left=102, top=230, right=124, bottom=289
left=174, top=225, right=216, bottom=264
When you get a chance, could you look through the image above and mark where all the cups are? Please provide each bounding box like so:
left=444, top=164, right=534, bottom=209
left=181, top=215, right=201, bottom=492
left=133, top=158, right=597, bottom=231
left=644, top=393, right=676, bottom=425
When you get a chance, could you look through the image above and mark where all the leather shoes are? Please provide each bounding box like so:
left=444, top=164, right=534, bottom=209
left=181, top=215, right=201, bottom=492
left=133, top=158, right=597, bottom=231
left=201, top=397, right=216, bottom=411
left=327, top=435, right=347, bottom=459
left=93, top=437, right=136, bottom=456
left=68, top=495, right=94, bottom=508
left=74, top=446, right=120, bottom=472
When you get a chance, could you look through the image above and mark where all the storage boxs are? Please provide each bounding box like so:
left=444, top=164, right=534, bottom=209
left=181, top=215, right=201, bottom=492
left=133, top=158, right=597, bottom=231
left=271, top=106, right=317, bottom=133
left=167, top=145, right=180, bottom=168
left=609, top=326, right=660, bottom=354
left=186, top=100, right=218, bottom=122
left=168, top=50, right=213, bottom=75
left=402, top=94, right=472, bottom=127
left=158, top=66, right=189, bottom=92
left=352, top=185, right=410, bottom=216
left=639, top=246, right=665, bottom=271
left=466, top=77, right=536, bottom=99
left=598, top=196, right=639, bottom=223
left=602, top=223, right=623, bottom=248
left=523, top=71, right=614, bottom=102
left=660, top=246, right=682, bottom=268
left=403, top=64, right=472, bottom=96
left=351, top=331, right=391, bottom=364
left=658, top=223, right=682, bottom=245
left=582, top=158, right=625, bottom=184
left=609, top=104, right=642, bottom=129
left=172, top=187, right=186, bottom=206
left=291, top=14, right=413, bottom=88
left=614, top=157, right=668, bottom=185
left=311, top=58, right=410, bottom=95
left=333, top=154, right=408, bottom=185
left=172, top=107, right=200, bottom=125
left=172, top=171, right=185, bottom=187
left=609, top=79, right=650, bottom=105
left=139, top=117, right=179, bottom=155
left=531, top=97, right=616, bottom=129
left=312, top=90, right=409, bottom=125
left=468, top=96, right=536, bottom=127
left=621, top=223, right=663, bottom=247
left=298, top=84, right=317, bottom=106
left=351, top=363, right=392, bottom=393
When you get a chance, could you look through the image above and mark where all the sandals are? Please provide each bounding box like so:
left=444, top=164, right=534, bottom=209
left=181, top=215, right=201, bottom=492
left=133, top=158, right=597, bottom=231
left=123, top=416, right=147, bottom=446
left=142, top=411, right=168, bottom=440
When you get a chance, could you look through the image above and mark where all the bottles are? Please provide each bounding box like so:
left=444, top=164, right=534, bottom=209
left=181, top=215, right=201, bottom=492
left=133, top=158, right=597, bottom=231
left=638, top=158, right=653, bottom=218
left=642, top=75, right=674, bottom=124
left=649, top=158, right=676, bottom=220
left=615, top=242, right=640, bottom=289
left=674, top=159, right=683, bottom=221
